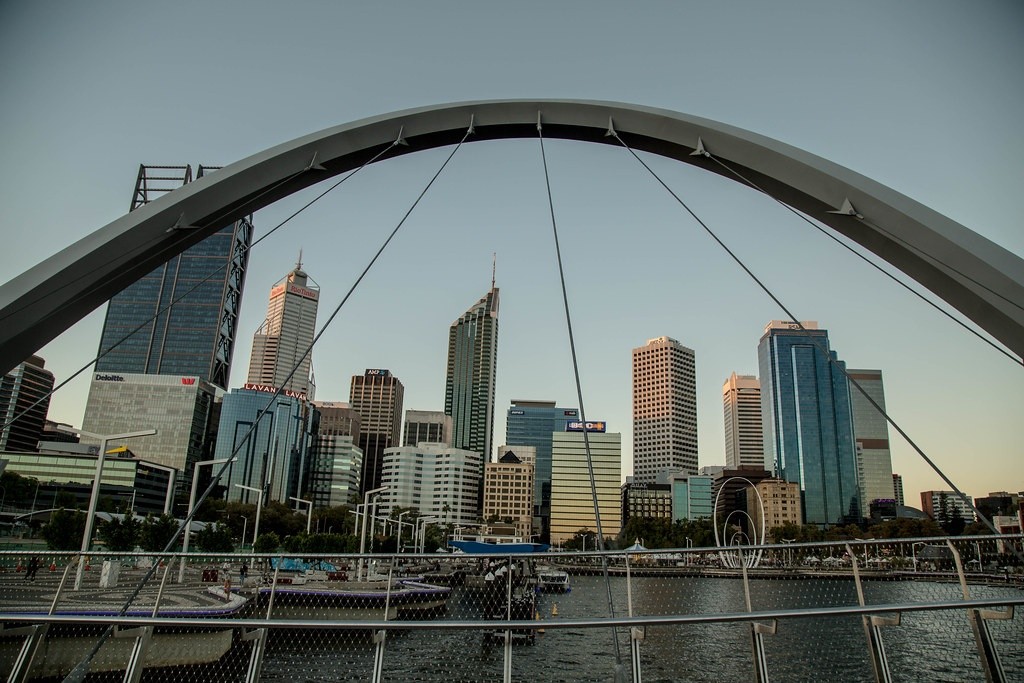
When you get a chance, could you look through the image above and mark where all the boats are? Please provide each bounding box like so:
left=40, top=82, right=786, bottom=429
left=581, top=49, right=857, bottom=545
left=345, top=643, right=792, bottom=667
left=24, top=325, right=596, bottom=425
left=536, top=570, right=571, bottom=593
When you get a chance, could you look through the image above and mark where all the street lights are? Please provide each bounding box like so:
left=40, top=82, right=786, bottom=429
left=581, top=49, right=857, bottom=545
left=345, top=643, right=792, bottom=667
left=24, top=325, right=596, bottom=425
left=235, top=483, right=263, bottom=569
left=452, top=526, right=467, bottom=552
left=413, top=515, right=435, bottom=554
left=685, top=536, right=689, bottom=566
left=855, top=537, right=875, bottom=569
left=359, top=486, right=389, bottom=553
left=289, top=496, right=313, bottom=535
left=396, top=511, right=410, bottom=554
left=239, top=515, right=248, bottom=549
left=73, top=428, right=159, bottom=591
left=580, top=533, right=588, bottom=561
left=782, top=538, right=796, bottom=566
left=177, top=455, right=238, bottom=584
left=688, top=539, right=694, bottom=565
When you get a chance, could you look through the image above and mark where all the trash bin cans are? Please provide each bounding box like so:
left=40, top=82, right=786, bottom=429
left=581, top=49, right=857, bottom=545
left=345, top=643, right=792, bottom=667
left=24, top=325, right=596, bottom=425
left=203, top=569, right=218, bottom=582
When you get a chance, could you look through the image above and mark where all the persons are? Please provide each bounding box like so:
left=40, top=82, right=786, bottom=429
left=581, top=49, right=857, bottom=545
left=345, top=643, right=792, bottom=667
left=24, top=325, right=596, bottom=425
left=387, top=566, right=411, bottom=578
left=223, top=559, right=232, bottom=592
left=240, top=561, right=248, bottom=587
left=24, top=550, right=40, bottom=582
